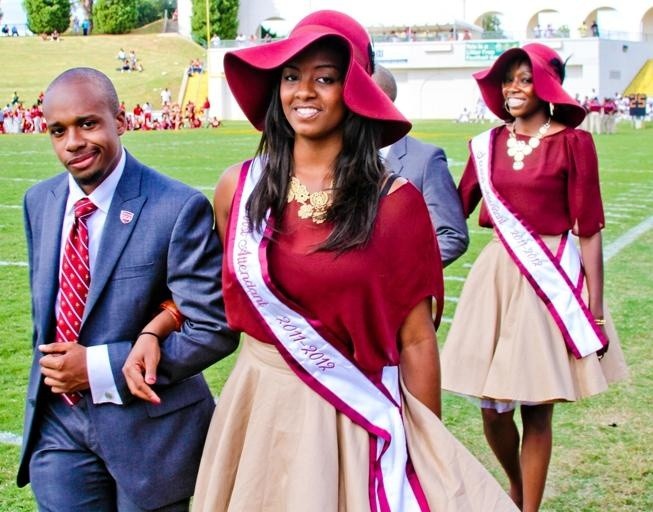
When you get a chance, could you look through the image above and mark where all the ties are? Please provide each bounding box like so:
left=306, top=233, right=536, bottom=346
left=52, top=197, right=98, bottom=408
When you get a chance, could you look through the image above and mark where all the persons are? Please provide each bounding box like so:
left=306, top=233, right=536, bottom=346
left=574, top=88, right=653, bottom=135
left=384, top=26, right=470, bottom=42
left=459, top=95, right=500, bottom=124
left=2, top=24, right=18, bottom=37
left=17, top=68, right=240, bottom=511
left=117, top=49, right=142, bottom=71
left=457, top=44, right=609, bottom=511
left=0, top=87, right=221, bottom=134
left=73, top=16, right=91, bottom=36
left=186, top=57, right=204, bottom=76
left=372, top=63, right=468, bottom=269
left=40, top=30, right=60, bottom=43
left=122, top=10, right=442, bottom=511
left=211, top=32, right=221, bottom=47
left=236, top=33, right=272, bottom=43
left=533, top=21, right=599, bottom=37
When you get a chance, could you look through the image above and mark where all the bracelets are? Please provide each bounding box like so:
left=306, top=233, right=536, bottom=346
left=592, top=316, right=606, bottom=328
left=138, top=332, right=160, bottom=338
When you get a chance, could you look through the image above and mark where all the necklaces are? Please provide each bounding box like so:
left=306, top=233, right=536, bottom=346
left=286, top=175, right=330, bottom=225
left=506, top=121, right=550, bottom=170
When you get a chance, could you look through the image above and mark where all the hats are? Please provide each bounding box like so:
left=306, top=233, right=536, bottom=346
left=223, top=10, right=413, bottom=150
left=472, top=42, right=586, bottom=129
